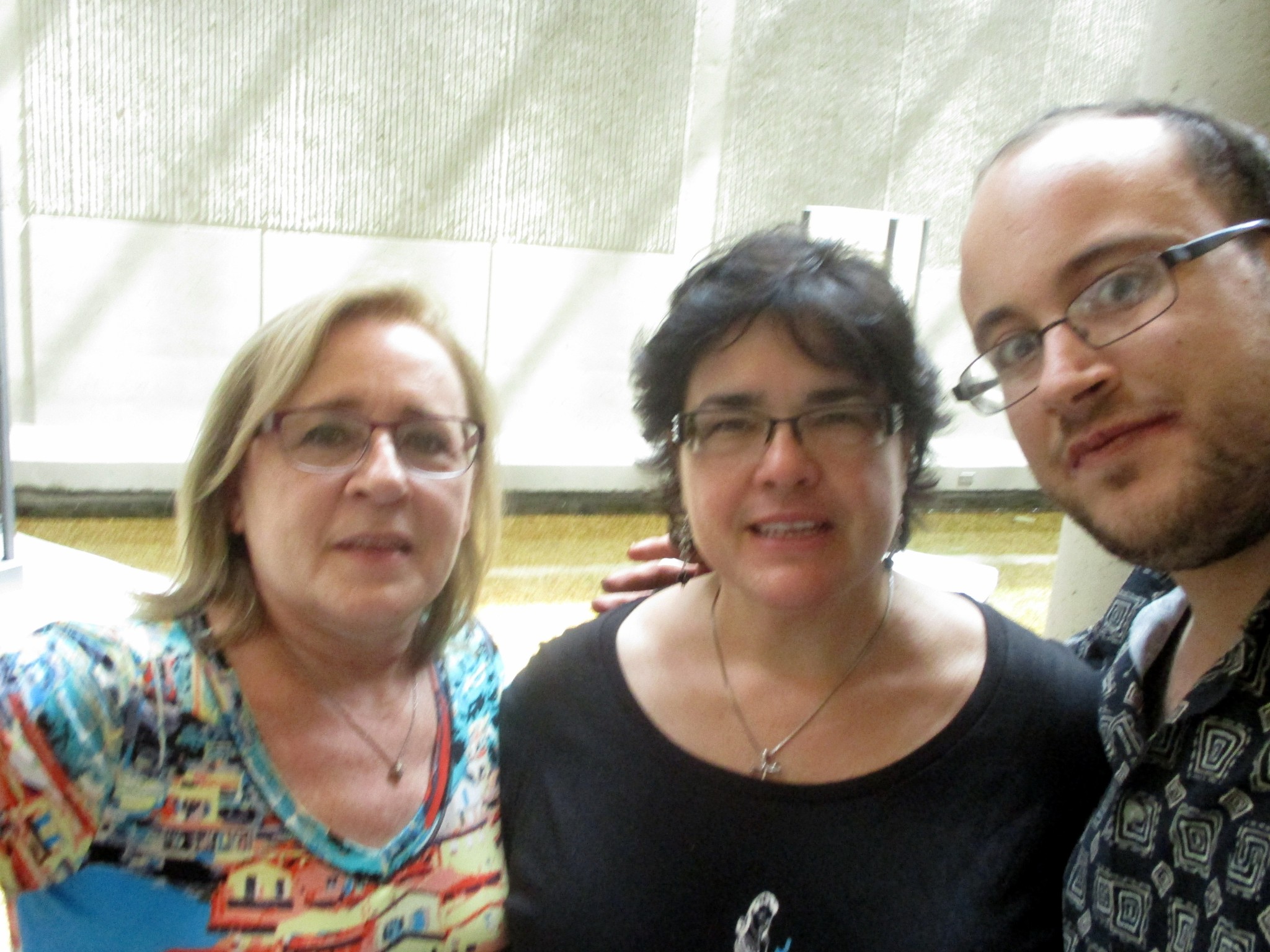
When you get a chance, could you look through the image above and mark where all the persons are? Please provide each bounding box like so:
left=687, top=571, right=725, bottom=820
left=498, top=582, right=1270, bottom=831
left=591, top=99, right=1270, bottom=952
left=0, top=285, right=508, bottom=952
left=499, top=229, right=1101, bottom=952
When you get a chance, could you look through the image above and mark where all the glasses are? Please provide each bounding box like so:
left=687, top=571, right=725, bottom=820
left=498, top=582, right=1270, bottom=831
left=953, top=220, right=1270, bottom=415
left=670, top=403, right=912, bottom=451
left=255, top=405, right=487, bottom=480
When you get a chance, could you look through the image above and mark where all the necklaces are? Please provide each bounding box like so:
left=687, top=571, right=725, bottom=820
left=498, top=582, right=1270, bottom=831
left=712, top=577, right=893, bottom=782
left=296, top=663, right=416, bottom=780
